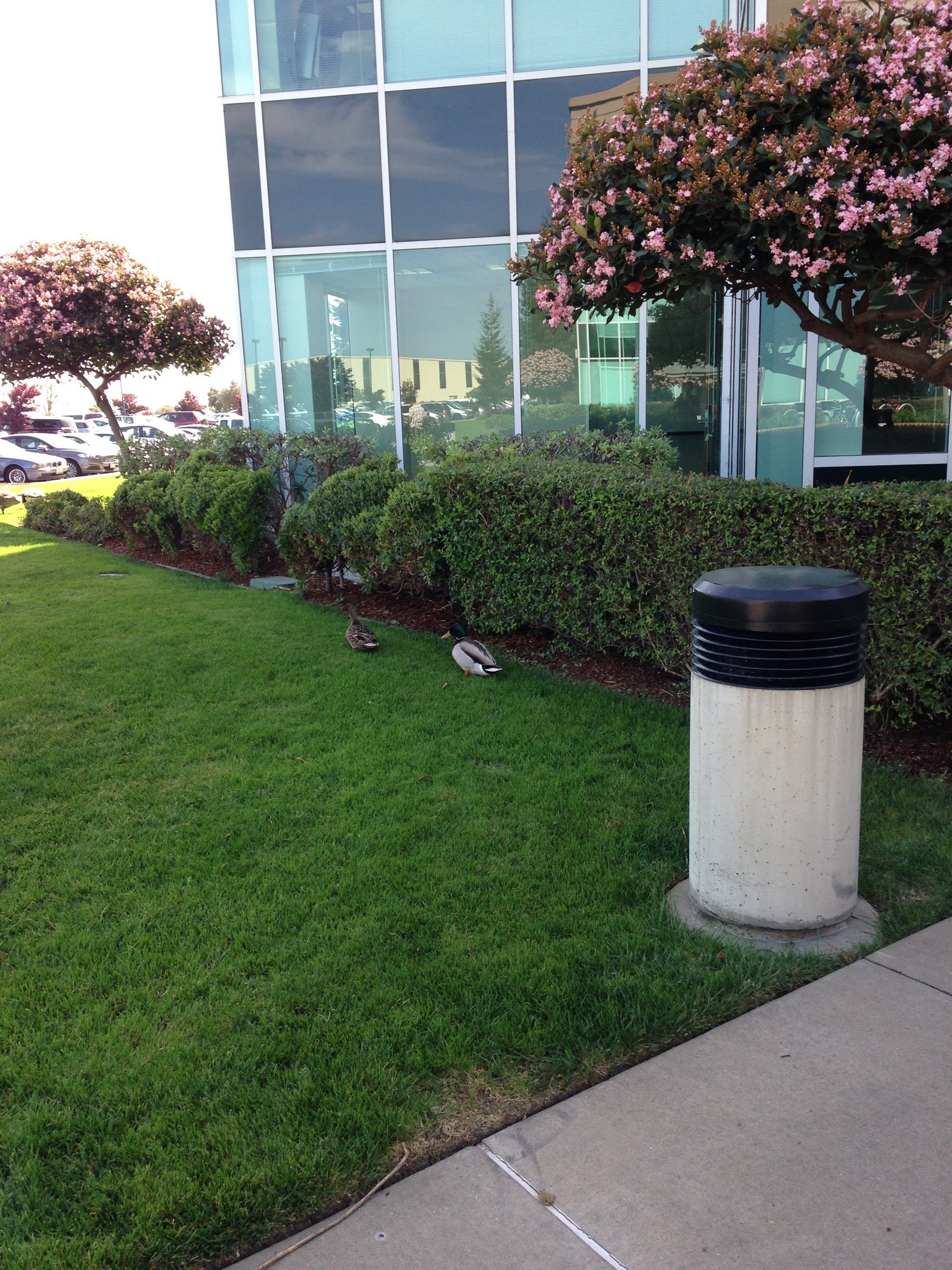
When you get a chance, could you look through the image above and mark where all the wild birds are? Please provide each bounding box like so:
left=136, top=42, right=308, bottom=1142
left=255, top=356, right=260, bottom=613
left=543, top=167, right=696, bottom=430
left=17, top=488, right=46, bottom=505
left=344, top=609, right=380, bottom=651
left=0, top=490, right=22, bottom=515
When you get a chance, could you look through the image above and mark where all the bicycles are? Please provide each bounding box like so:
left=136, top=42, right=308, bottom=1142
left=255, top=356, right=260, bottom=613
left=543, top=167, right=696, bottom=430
left=784, top=392, right=918, bottom=427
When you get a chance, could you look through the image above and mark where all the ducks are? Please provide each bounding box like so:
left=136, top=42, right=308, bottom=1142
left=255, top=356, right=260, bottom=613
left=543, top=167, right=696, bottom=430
left=440, top=625, right=503, bottom=676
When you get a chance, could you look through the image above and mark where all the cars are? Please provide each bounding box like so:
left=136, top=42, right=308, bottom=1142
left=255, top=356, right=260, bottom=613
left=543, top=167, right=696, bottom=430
left=0, top=439, right=70, bottom=482
left=0, top=395, right=518, bottom=478
